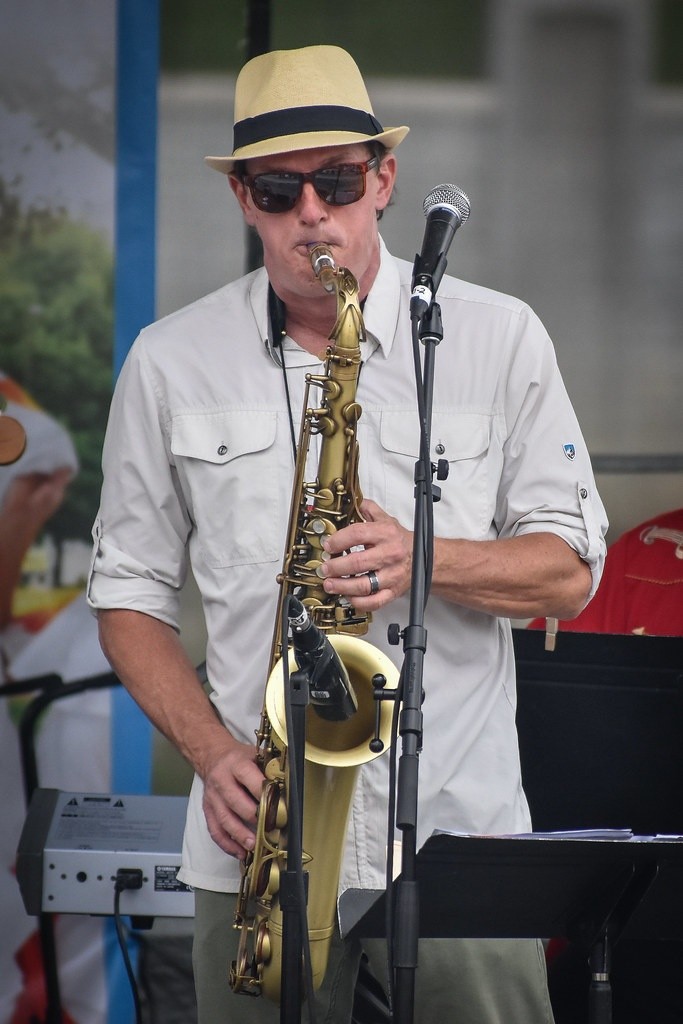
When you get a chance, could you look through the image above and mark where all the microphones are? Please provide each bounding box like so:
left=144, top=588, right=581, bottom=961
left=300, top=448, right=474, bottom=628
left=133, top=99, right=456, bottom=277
left=287, top=597, right=358, bottom=721
left=409, top=184, right=471, bottom=320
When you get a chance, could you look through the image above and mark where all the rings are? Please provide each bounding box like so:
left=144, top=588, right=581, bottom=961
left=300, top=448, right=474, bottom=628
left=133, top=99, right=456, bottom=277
left=365, top=573, right=379, bottom=595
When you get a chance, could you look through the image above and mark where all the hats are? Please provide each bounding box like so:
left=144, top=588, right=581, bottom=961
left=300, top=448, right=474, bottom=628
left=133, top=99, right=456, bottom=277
left=203, top=45, right=410, bottom=175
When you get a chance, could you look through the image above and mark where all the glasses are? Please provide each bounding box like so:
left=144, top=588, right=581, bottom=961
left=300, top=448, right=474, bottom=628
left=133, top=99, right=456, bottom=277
left=241, top=156, right=377, bottom=213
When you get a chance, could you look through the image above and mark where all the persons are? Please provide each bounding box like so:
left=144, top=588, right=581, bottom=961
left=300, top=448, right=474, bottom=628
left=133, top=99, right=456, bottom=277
left=88, top=44, right=611, bottom=1022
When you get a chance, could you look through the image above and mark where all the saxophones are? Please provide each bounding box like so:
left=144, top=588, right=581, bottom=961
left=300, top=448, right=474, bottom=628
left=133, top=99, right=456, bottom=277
left=231, top=234, right=433, bottom=999
